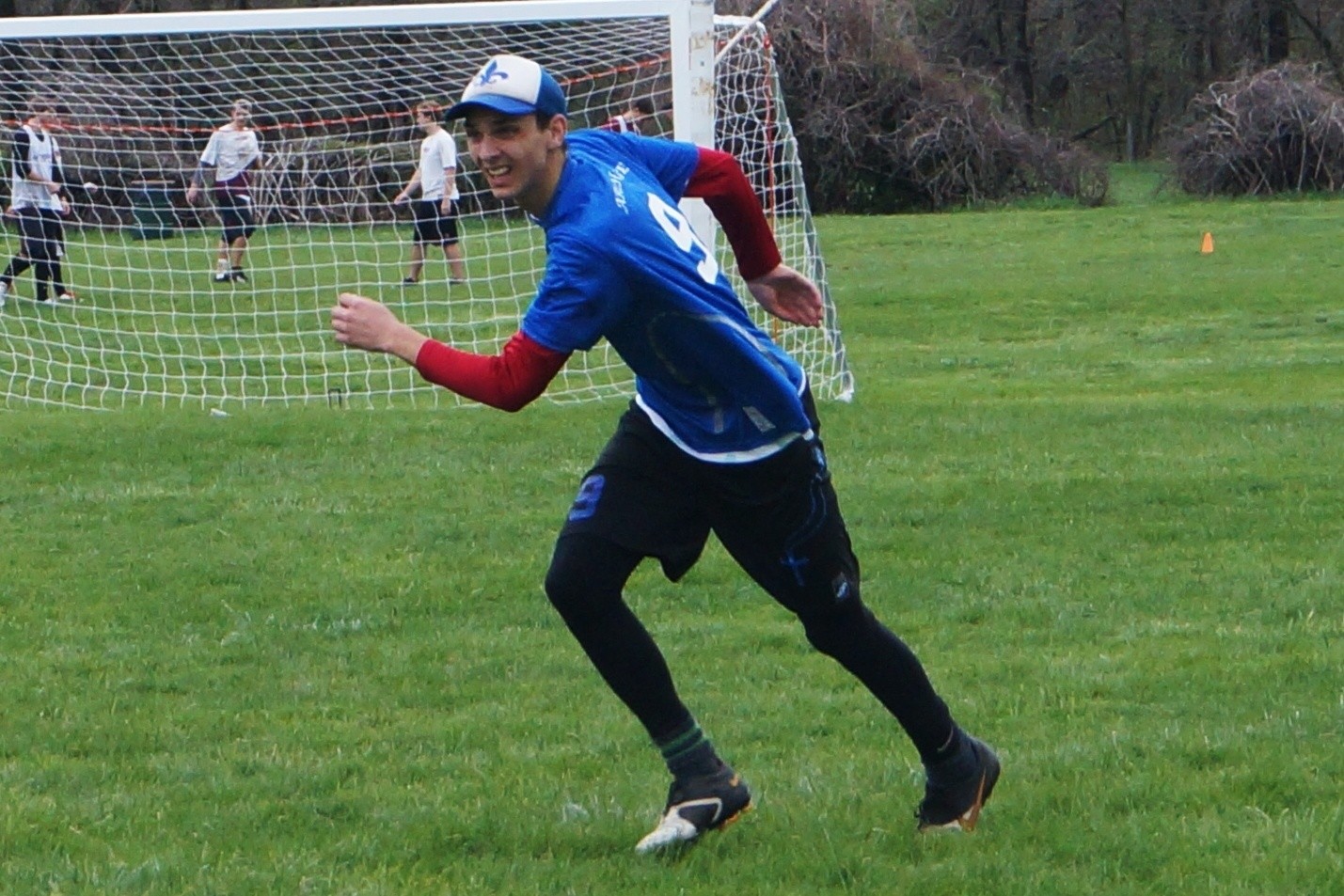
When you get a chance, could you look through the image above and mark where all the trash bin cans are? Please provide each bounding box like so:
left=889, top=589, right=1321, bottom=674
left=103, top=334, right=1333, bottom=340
left=132, top=180, right=175, bottom=240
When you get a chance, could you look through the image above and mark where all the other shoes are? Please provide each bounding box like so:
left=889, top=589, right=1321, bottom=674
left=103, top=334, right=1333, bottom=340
left=449, top=276, right=467, bottom=285
left=231, top=266, right=248, bottom=285
left=44, top=298, right=52, bottom=304
left=60, top=291, right=84, bottom=302
left=393, top=276, right=420, bottom=288
left=0, top=281, right=8, bottom=307
left=214, top=261, right=230, bottom=282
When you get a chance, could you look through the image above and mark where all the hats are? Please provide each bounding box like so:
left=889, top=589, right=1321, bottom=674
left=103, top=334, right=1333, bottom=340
left=442, top=53, right=575, bottom=124
left=29, top=96, right=52, bottom=115
left=233, top=99, right=253, bottom=115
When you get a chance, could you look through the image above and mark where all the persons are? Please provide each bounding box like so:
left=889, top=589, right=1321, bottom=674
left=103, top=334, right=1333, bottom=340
left=185, top=99, right=263, bottom=283
left=0, top=97, right=98, bottom=307
left=330, top=53, right=1001, bottom=856
left=600, top=98, right=674, bottom=134
left=394, top=99, right=464, bottom=286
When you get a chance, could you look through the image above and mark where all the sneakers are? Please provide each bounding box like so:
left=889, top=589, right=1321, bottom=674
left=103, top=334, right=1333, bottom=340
left=634, top=755, right=752, bottom=861
left=912, top=737, right=1000, bottom=834
